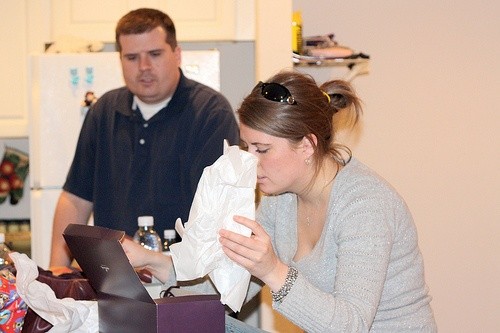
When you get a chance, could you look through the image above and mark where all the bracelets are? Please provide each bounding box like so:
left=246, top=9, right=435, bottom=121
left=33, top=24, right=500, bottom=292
left=270, top=266, right=298, bottom=302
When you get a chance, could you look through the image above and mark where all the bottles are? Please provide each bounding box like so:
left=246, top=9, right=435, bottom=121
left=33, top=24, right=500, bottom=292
left=133, top=215, right=163, bottom=252
left=162, top=229, right=178, bottom=252
left=0, top=232, right=13, bottom=269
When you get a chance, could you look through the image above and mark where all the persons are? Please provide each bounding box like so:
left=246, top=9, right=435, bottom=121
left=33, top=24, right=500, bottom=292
left=49, top=8, right=243, bottom=277
left=121, top=72, right=437, bottom=333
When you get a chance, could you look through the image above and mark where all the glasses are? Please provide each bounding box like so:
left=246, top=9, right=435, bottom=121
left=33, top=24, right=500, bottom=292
left=254, top=81, right=298, bottom=105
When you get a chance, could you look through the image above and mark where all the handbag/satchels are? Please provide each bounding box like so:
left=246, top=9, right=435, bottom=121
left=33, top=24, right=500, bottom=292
left=0, top=261, right=96, bottom=333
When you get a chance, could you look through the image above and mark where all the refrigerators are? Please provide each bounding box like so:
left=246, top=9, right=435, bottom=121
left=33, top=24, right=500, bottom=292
left=26, top=51, right=220, bottom=269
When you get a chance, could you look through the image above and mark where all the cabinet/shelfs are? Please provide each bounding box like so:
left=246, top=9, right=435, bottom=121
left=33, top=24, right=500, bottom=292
left=0, top=0, right=257, bottom=137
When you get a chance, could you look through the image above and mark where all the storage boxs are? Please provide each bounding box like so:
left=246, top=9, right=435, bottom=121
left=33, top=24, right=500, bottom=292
left=62, top=224, right=225, bottom=333
